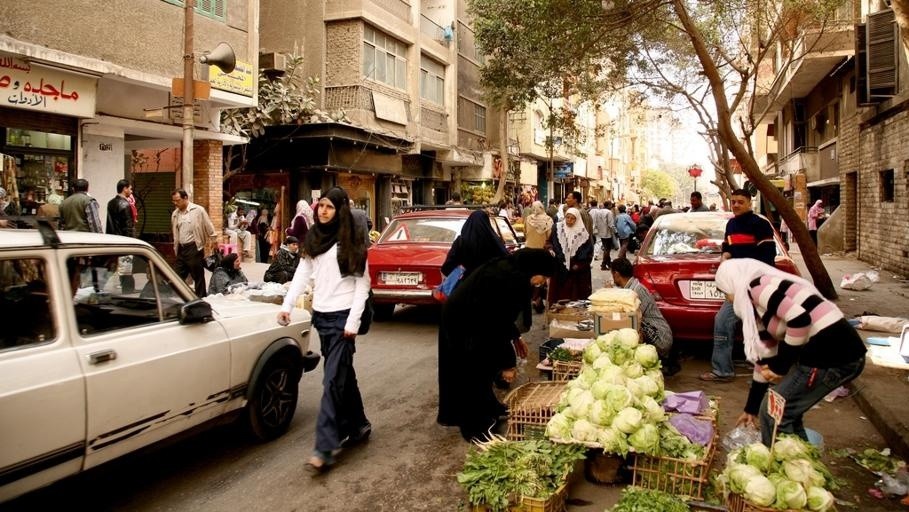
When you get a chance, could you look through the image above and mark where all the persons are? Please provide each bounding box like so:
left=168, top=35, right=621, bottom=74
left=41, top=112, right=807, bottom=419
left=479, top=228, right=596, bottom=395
left=276, top=187, right=371, bottom=475
left=699, top=190, right=777, bottom=382
left=431, top=209, right=554, bottom=447
left=611, top=257, right=682, bottom=376
left=170, top=188, right=214, bottom=297
left=58, top=179, right=102, bottom=288
left=493, top=191, right=716, bottom=313
left=716, top=259, right=869, bottom=450
left=446, top=192, right=467, bottom=210
left=1, top=188, right=64, bottom=284
left=779, top=200, right=825, bottom=251
left=208, top=199, right=375, bottom=335
left=105, top=179, right=134, bottom=293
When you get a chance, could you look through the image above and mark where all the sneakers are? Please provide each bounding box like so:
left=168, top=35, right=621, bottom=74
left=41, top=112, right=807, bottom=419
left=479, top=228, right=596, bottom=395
left=699, top=371, right=735, bottom=381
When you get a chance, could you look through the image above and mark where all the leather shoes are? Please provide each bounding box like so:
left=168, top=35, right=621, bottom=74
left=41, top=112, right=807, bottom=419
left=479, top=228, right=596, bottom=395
left=303, top=461, right=326, bottom=473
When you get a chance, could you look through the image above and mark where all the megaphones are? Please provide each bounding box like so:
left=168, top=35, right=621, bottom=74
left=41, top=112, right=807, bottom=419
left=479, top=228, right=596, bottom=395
left=200, top=41, right=236, bottom=73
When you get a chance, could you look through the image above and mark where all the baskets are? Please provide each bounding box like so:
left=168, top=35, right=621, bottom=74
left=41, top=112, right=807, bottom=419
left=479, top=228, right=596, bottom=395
left=633, top=396, right=722, bottom=502
left=473, top=481, right=567, bottom=512
left=552, top=359, right=581, bottom=380
left=729, top=491, right=800, bottom=512
left=547, top=308, right=590, bottom=323
left=504, top=379, right=568, bottom=439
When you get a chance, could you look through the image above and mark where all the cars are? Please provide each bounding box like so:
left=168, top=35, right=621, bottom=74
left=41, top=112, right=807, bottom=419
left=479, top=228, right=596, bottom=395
left=0, top=212, right=323, bottom=505
left=364, top=204, right=526, bottom=324
left=631, top=209, right=805, bottom=348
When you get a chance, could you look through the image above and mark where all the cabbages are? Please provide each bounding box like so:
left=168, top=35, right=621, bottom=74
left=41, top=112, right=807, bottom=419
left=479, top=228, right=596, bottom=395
left=544, top=327, right=665, bottom=456
left=633, top=387, right=720, bottom=498
left=713, top=426, right=835, bottom=512
